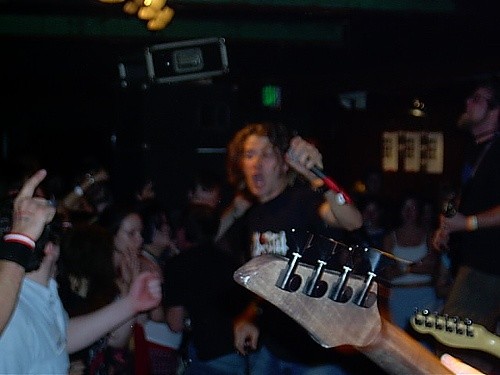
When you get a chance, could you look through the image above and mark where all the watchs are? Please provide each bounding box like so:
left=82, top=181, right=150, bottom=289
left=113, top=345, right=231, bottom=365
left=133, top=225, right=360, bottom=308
left=86, top=173, right=96, bottom=186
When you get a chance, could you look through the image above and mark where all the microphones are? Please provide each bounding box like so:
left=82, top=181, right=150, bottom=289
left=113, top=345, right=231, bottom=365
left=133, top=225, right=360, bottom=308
left=279, top=135, right=352, bottom=204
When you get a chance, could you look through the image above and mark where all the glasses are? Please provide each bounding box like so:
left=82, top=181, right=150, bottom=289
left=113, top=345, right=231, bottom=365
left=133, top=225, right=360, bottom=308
left=474, top=94, right=489, bottom=103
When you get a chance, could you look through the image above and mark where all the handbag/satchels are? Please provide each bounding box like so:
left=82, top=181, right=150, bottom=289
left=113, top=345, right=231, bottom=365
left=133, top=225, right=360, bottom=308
left=144, top=317, right=182, bottom=350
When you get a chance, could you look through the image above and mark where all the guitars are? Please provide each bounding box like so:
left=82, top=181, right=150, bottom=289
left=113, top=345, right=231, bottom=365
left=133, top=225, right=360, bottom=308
left=412, top=305, right=499, bottom=356
left=233, top=251, right=457, bottom=375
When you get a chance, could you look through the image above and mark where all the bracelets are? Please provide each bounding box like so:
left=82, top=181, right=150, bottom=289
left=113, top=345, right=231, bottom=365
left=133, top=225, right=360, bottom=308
left=310, top=178, right=329, bottom=195
left=74, top=186, right=84, bottom=197
left=1, top=233, right=36, bottom=268
left=466, top=216, right=479, bottom=232
left=253, top=297, right=263, bottom=309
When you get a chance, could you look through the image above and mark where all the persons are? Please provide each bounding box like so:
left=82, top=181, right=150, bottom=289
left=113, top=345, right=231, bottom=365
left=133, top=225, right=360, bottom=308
left=430, top=80, right=500, bottom=375
left=0, top=122, right=445, bottom=375
left=193, top=123, right=364, bottom=374
